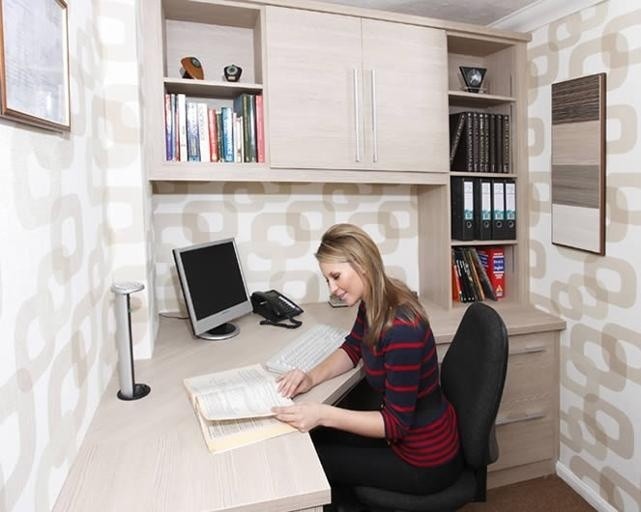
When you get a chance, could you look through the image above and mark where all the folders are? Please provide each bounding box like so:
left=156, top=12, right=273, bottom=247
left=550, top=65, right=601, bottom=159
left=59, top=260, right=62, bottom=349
left=451, top=176, right=516, bottom=242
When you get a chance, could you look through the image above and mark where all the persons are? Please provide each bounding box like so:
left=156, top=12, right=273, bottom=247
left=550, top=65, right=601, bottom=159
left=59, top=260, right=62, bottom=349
left=271, top=223, right=462, bottom=495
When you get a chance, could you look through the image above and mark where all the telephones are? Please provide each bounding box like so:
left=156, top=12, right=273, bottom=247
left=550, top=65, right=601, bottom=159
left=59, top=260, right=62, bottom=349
left=251, top=290, right=304, bottom=322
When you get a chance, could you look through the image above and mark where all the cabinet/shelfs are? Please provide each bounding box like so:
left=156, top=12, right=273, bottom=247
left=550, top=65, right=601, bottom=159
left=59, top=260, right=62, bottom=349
left=136, top=0, right=264, bottom=182
left=415, top=17, right=532, bottom=305
left=253, top=1, right=452, bottom=184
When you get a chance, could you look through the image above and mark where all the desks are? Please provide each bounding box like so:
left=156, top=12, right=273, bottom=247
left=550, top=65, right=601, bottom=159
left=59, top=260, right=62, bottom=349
left=50, top=300, right=566, bottom=511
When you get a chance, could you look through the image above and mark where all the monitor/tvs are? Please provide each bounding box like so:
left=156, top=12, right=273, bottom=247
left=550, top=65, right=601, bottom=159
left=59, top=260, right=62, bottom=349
left=171, top=236, right=254, bottom=340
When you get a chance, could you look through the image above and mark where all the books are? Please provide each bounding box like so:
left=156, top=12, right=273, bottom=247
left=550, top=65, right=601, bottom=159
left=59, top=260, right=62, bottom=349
left=450, top=112, right=509, bottom=174
left=452, top=247, right=505, bottom=304
left=164, top=87, right=264, bottom=164
left=182, top=363, right=295, bottom=453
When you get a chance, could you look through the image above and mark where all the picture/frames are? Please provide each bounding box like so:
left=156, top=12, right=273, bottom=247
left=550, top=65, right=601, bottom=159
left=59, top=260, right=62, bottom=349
left=549, top=70, right=610, bottom=258
left=0, top=1, right=75, bottom=140
